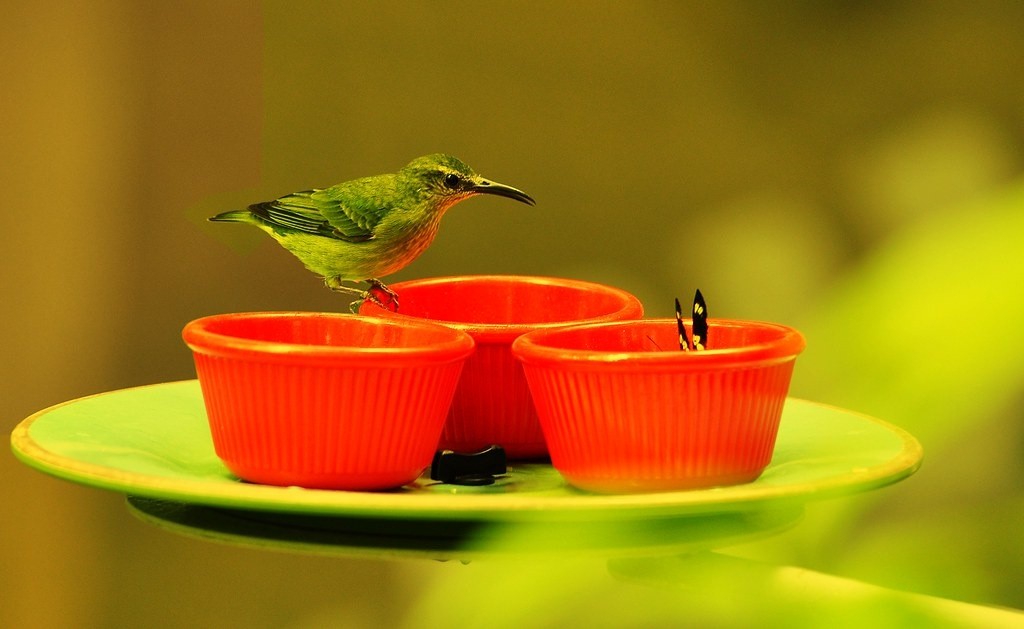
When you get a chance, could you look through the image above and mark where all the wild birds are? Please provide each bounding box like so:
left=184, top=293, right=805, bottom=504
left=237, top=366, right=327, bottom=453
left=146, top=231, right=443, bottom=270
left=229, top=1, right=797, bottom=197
left=206, top=153, right=535, bottom=315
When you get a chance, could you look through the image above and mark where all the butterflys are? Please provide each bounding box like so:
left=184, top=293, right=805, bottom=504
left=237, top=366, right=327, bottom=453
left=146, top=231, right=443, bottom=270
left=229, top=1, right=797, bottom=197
left=647, top=290, right=707, bottom=350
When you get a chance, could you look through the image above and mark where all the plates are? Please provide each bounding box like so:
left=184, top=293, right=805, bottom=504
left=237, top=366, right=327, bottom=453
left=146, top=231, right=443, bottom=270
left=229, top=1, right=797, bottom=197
left=9, top=375, right=925, bottom=548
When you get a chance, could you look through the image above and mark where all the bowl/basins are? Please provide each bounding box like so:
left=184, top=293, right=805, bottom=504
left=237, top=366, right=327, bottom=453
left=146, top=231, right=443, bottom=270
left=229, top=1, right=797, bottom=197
left=181, top=310, right=474, bottom=492
left=512, top=318, right=807, bottom=496
left=357, top=275, right=647, bottom=465
